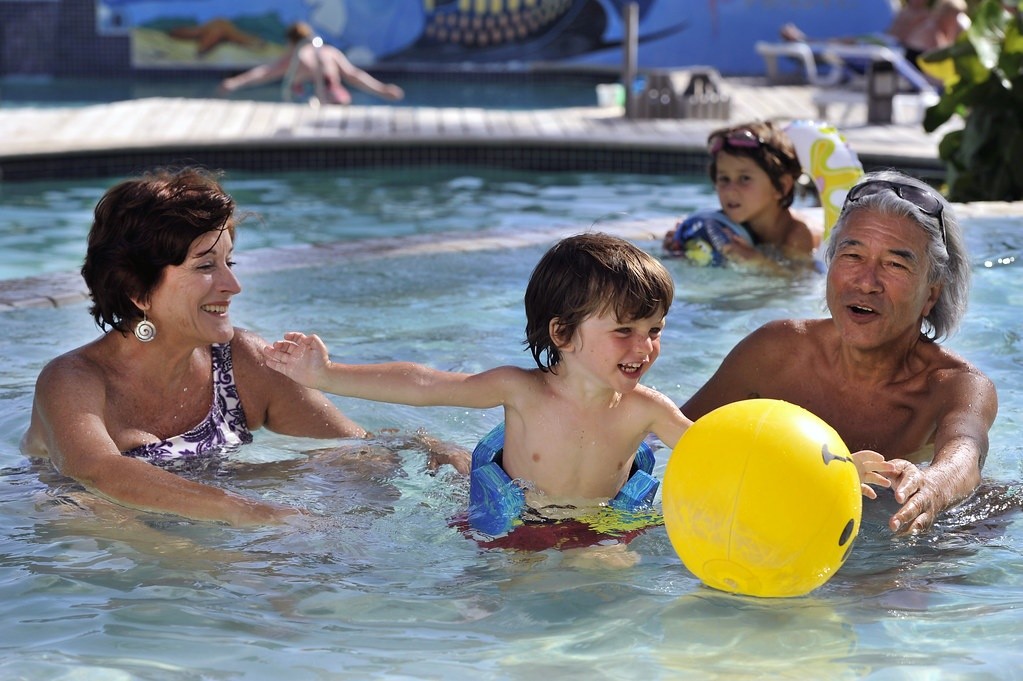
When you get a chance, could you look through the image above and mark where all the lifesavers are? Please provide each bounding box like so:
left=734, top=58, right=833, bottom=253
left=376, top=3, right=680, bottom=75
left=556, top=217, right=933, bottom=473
left=771, top=117, right=866, bottom=244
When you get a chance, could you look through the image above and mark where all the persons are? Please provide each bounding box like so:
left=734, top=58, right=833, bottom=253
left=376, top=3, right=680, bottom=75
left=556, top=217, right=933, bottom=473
left=17, top=168, right=471, bottom=570
left=217, top=21, right=407, bottom=111
left=259, top=228, right=900, bottom=602
left=771, top=0, right=975, bottom=104
left=670, top=173, right=999, bottom=554
left=656, top=116, right=825, bottom=296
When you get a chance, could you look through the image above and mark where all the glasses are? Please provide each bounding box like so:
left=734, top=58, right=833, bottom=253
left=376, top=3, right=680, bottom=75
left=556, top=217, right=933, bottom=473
left=842, top=180, right=949, bottom=254
left=707, top=126, right=760, bottom=154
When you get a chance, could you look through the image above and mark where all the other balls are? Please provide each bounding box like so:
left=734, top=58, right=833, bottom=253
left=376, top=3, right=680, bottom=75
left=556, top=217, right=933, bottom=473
left=662, top=399, right=862, bottom=597
